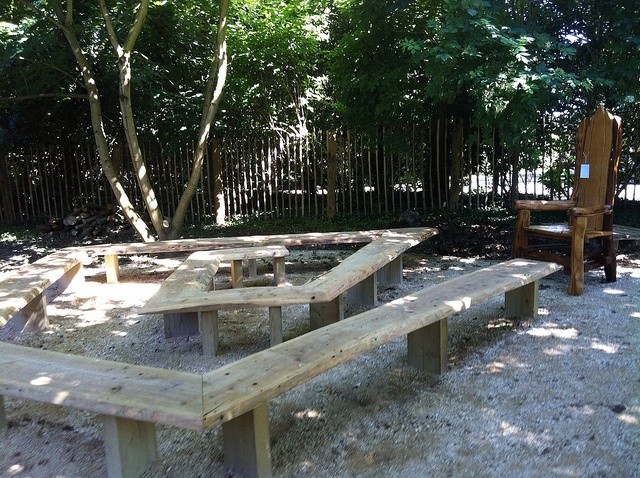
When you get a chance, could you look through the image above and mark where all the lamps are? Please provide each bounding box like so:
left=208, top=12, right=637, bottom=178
left=515, top=106, right=624, bottom=294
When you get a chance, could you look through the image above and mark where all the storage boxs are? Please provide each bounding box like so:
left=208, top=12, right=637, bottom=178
left=0, top=227, right=565, bottom=478
